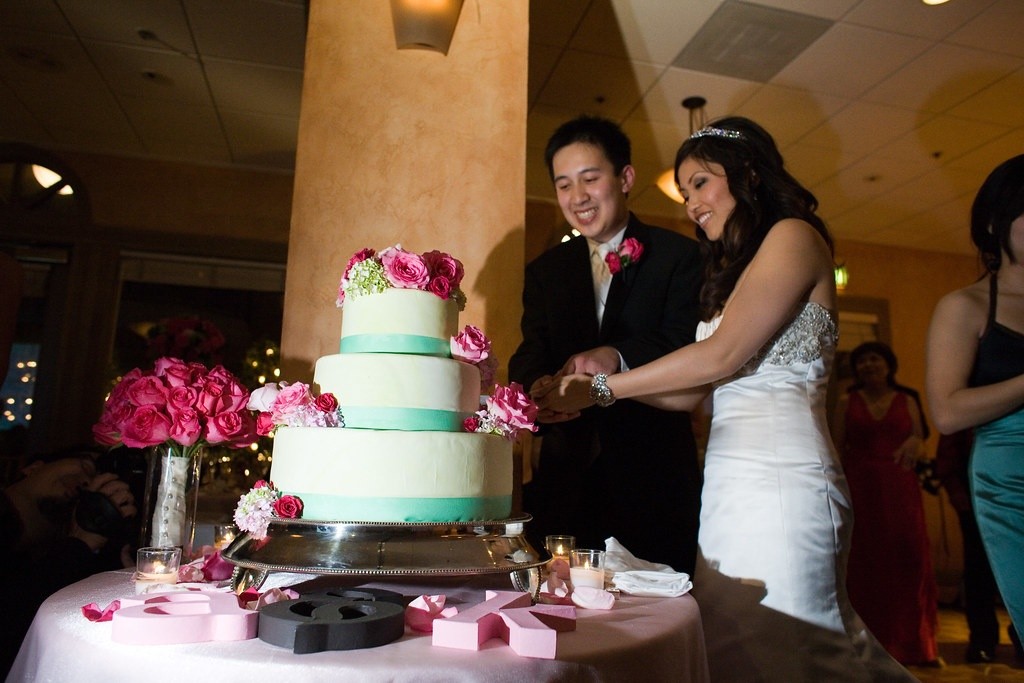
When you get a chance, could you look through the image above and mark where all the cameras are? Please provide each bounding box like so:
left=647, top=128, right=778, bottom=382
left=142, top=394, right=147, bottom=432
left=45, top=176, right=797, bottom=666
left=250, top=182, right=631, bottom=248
left=94, top=450, right=147, bottom=546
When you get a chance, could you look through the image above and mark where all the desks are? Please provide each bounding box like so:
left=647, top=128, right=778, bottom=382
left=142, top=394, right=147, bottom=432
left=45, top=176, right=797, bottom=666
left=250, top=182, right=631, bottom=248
left=2, top=558, right=712, bottom=683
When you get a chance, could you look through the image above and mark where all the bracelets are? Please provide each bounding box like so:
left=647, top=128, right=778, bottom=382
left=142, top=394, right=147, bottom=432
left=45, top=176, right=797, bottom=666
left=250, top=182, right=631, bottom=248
left=589, top=373, right=616, bottom=408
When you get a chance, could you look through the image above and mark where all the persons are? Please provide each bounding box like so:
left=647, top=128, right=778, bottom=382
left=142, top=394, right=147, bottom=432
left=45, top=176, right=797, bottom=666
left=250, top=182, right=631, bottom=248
left=831, top=341, right=949, bottom=672
left=508, top=112, right=712, bottom=593
left=530, top=114, right=925, bottom=683
left=926, top=152, right=1024, bottom=673
left=0, top=445, right=138, bottom=683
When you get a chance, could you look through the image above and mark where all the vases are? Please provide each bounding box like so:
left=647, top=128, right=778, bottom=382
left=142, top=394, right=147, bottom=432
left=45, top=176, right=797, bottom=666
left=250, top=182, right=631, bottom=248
left=138, top=446, right=200, bottom=576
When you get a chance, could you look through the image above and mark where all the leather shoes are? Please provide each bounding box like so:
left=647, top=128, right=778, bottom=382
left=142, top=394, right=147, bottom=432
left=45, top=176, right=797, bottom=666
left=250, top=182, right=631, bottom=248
left=965, top=643, right=995, bottom=662
left=1008, top=623, right=1024, bottom=665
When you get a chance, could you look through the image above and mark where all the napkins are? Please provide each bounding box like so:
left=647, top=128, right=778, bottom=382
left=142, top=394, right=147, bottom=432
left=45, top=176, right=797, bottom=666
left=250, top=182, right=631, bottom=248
left=600, top=537, right=692, bottom=598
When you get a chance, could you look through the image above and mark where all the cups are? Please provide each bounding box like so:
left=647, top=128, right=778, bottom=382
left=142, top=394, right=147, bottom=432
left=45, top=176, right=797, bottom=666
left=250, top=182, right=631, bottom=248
left=570, top=550, right=606, bottom=588
left=545, top=535, right=574, bottom=572
left=135, top=547, right=182, bottom=585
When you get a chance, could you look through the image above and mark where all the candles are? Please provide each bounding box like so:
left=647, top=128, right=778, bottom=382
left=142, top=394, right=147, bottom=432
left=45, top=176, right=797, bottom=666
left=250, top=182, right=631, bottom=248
left=567, top=548, right=605, bottom=591
left=214, top=523, right=238, bottom=549
left=133, top=547, right=182, bottom=589
left=544, top=534, right=577, bottom=566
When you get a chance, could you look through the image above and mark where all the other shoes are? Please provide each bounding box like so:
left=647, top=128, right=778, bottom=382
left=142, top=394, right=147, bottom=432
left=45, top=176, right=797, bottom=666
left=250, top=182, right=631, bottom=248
left=926, top=655, right=946, bottom=673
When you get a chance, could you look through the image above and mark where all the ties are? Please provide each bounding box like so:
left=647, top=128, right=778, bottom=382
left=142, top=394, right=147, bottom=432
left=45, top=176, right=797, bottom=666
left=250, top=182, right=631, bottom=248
left=595, top=244, right=613, bottom=331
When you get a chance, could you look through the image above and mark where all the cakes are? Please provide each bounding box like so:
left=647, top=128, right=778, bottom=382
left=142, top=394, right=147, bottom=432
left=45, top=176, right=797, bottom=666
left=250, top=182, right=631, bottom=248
left=246, top=243, right=540, bottom=523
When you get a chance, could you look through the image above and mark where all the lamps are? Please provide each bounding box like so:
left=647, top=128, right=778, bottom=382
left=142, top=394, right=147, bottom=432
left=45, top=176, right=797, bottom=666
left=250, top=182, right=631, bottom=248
left=390, top=0, right=464, bottom=55
left=655, top=94, right=707, bottom=205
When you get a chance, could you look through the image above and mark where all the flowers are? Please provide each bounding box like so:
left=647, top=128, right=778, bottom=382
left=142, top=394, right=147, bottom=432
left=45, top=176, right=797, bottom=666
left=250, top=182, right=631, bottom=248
left=334, top=245, right=468, bottom=311
left=233, top=480, right=305, bottom=537
left=463, top=378, right=540, bottom=438
left=448, top=323, right=498, bottom=390
left=605, top=237, right=647, bottom=286
left=141, top=314, right=225, bottom=367
left=90, top=357, right=259, bottom=456
left=247, top=381, right=344, bottom=438
